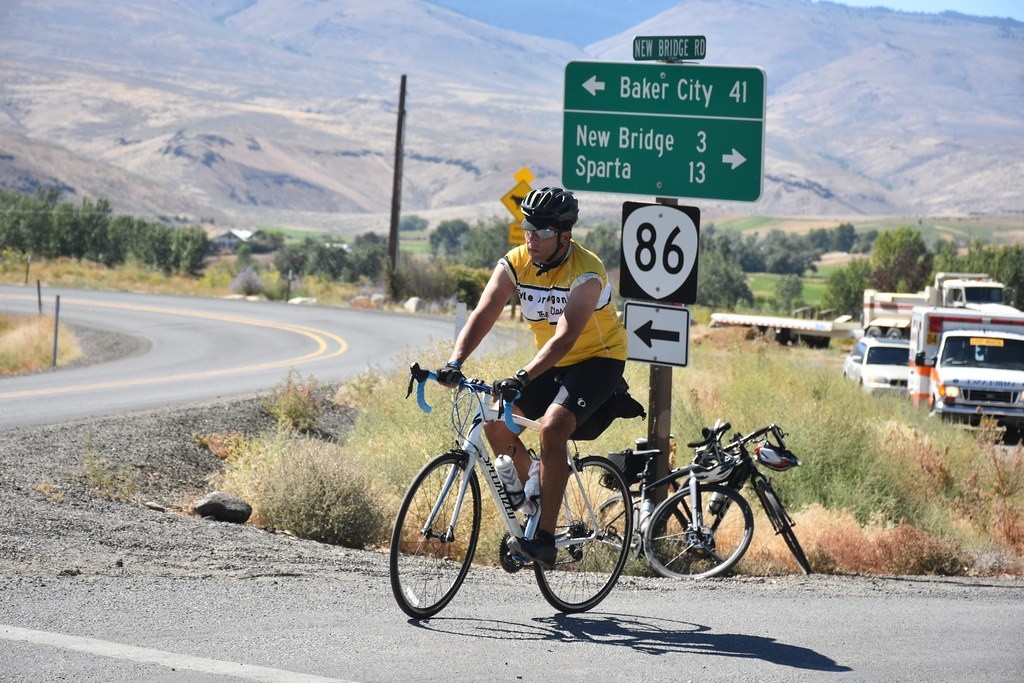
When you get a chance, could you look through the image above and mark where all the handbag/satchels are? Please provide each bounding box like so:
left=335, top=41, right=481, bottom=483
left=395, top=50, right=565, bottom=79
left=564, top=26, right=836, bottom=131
left=599, top=448, right=645, bottom=491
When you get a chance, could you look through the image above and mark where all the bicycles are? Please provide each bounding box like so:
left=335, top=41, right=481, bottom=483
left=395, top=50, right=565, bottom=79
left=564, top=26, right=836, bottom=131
left=581, top=420, right=811, bottom=581
left=389, top=362, right=634, bottom=620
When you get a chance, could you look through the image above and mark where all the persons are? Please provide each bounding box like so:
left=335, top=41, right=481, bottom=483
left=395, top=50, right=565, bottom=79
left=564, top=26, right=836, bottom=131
left=437, top=187, right=629, bottom=568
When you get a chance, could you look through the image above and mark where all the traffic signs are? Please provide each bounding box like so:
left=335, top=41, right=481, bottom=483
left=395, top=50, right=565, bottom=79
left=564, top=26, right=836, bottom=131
left=561, top=61, right=768, bottom=204
left=619, top=201, right=702, bottom=367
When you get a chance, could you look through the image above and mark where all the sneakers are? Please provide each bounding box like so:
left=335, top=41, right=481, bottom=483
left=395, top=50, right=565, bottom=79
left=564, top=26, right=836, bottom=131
left=510, top=531, right=557, bottom=570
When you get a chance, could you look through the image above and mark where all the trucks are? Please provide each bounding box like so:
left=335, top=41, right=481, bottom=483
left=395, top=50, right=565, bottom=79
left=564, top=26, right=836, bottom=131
left=907, top=306, right=1024, bottom=444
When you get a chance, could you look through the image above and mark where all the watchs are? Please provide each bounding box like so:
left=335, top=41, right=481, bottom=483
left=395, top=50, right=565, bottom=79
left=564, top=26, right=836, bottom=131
left=516, top=369, right=531, bottom=387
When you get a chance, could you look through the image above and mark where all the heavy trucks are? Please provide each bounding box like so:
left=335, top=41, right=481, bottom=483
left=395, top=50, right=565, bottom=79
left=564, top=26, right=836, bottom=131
left=709, top=273, right=1024, bottom=341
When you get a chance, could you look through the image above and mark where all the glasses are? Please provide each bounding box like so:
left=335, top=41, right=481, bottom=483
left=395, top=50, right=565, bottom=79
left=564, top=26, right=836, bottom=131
left=522, top=229, right=561, bottom=239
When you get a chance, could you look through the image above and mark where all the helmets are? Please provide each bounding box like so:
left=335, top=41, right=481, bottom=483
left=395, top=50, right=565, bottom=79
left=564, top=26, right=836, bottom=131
left=754, top=440, right=802, bottom=471
left=691, top=450, right=736, bottom=482
left=520, top=186, right=579, bottom=230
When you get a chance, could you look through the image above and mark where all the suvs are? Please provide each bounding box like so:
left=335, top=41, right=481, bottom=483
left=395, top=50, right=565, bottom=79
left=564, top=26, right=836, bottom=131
left=843, top=337, right=911, bottom=398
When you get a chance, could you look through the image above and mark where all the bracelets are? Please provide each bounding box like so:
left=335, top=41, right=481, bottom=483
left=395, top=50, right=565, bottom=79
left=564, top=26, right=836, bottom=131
left=445, top=361, right=461, bottom=369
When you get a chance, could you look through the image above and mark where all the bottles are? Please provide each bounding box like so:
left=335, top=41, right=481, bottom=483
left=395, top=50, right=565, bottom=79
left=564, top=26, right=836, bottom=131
left=494, top=455, right=527, bottom=508
left=518, top=458, right=542, bottom=515
left=706, top=482, right=729, bottom=514
left=640, top=498, right=654, bottom=533
